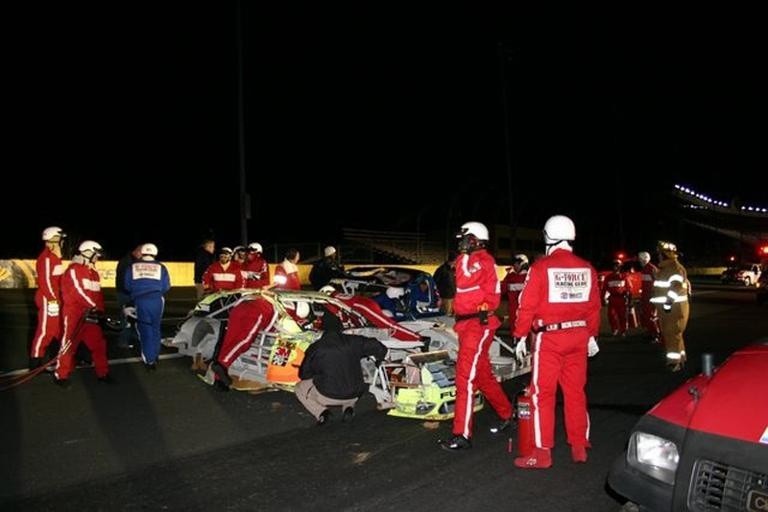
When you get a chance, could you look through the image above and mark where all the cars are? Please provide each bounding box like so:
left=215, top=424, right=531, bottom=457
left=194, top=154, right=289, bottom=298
left=623, top=336, right=768, bottom=510
left=719, top=261, right=763, bottom=286
left=169, top=284, right=486, bottom=423
left=319, top=262, right=445, bottom=323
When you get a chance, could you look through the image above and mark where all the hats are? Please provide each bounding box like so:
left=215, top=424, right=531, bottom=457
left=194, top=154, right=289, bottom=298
left=140, top=243, right=158, bottom=257
left=321, top=306, right=344, bottom=330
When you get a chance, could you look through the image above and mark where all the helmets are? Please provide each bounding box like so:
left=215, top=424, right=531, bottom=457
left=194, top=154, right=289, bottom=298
left=656, top=242, right=678, bottom=260
left=639, top=252, right=651, bottom=262
left=456, top=222, right=490, bottom=240
left=220, top=242, right=337, bottom=259
left=42, top=226, right=67, bottom=240
left=79, top=240, right=104, bottom=257
left=514, top=254, right=529, bottom=264
left=545, top=215, right=576, bottom=245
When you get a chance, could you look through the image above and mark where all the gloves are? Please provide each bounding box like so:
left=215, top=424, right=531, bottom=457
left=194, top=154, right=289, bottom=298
left=588, top=336, right=600, bottom=357
left=515, top=336, right=527, bottom=359
left=538, top=320, right=587, bottom=332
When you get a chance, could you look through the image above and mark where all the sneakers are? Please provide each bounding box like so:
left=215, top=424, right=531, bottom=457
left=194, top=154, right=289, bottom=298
left=436, top=418, right=587, bottom=467
left=315, top=411, right=335, bottom=429
left=54, top=379, right=72, bottom=390
left=97, top=375, right=119, bottom=386
left=343, top=408, right=355, bottom=422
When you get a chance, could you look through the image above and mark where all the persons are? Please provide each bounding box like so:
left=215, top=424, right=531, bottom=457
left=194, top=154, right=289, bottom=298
left=437, top=221, right=514, bottom=453
left=194, top=235, right=302, bottom=305
left=116, top=243, right=171, bottom=367
left=295, top=311, right=388, bottom=427
left=512, top=215, right=603, bottom=470
left=308, top=245, right=458, bottom=352
left=499, top=254, right=530, bottom=359
left=209, top=295, right=314, bottom=392
left=30, top=226, right=93, bottom=375
left=50, top=240, right=120, bottom=389
left=599, top=239, right=694, bottom=373
